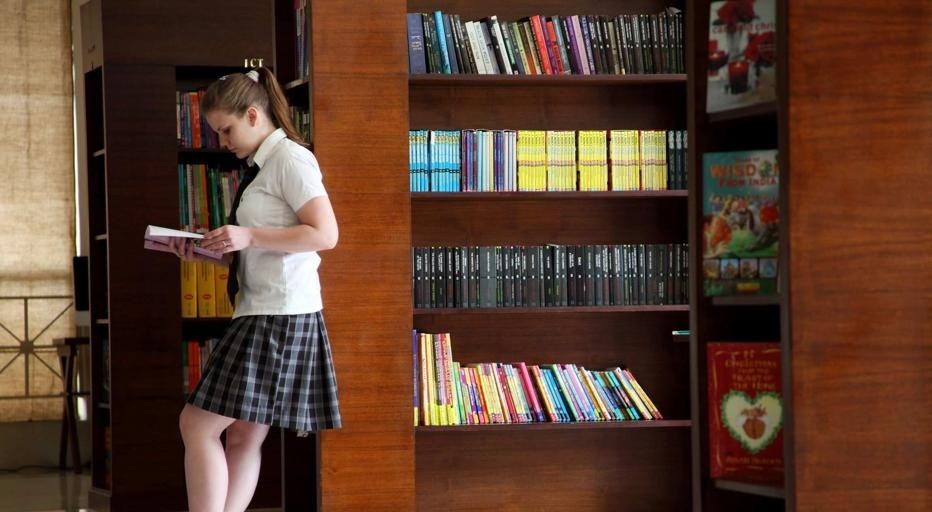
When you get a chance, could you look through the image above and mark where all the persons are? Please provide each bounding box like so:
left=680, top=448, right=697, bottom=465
left=169, top=66, right=345, bottom=512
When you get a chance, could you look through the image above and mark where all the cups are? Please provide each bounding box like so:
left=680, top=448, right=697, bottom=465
left=709, top=52, right=726, bottom=71
left=728, top=61, right=748, bottom=94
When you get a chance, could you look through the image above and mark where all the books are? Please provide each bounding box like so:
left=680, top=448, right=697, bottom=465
left=413, top=244, right=689, bottom=309
left=706, top=339, right=787, bottom=484
left=142, top=223, right=223, bottom=261
left=408, top=130, right=688, bottom=192
left=175, top=90, right=313, bottom=397
left=293, top=1, right=309, bottom=80
left=704, top=1, right=779, bottom=114
left=412, top=329, right=663, bottom=427
left=699, top=148, right=784, bottom=300
left=406, top=6, right=686, bottom=74
